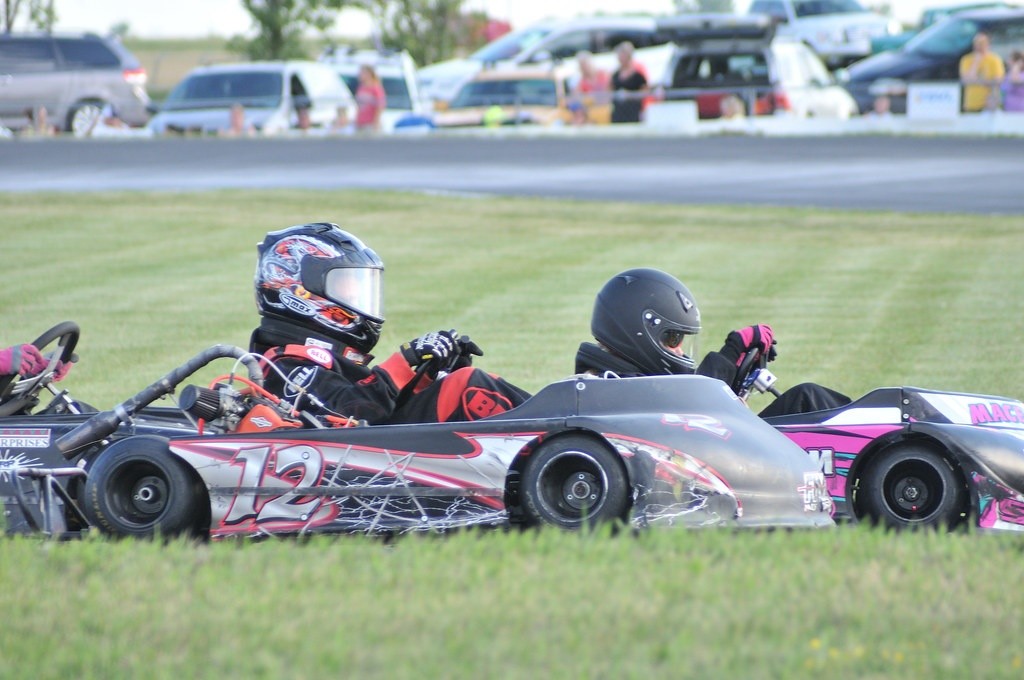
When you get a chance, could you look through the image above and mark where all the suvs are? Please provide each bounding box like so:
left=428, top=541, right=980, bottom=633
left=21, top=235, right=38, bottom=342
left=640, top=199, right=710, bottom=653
left=149, top=0, right=1024, bottom=134
left=0, top=33, right=149, bottom=138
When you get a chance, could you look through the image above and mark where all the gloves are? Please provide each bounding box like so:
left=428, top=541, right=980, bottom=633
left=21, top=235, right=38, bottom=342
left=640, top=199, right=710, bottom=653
left=726, top=324, right=777, bottom=368
left=0, top=344, right=43, bottom=375
left=22, top=350, right=79, bottom=382
left=399, top=329, right=484, bottom=374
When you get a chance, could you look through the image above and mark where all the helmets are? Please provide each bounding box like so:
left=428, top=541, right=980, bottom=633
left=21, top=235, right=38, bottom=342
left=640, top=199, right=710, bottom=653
left=254, top=223, right=385, bottom=353
left=591, top=268, right=702, bottom=376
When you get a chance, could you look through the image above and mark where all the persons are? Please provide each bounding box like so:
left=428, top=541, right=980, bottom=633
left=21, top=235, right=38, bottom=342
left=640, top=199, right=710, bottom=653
left=609, top=42, right=648, bottom=123
left=291, top=101, right=322, bottom=129
left=959, top=32, right=1004, bottom=112
left=221, top=103, right=256, bottom=137
left=1000, top=53, right=1024, bottom=111
left=327, top=103, right=356, bottom=129
left=718, top=96, right=746, bottom=120
left=0, top=344, right=80, bottom=415
left=353, top=65, right=387, bottom=130
left=574, top=268, right=777, bottom=400
left=573, top=51, right=608, bottom=93
left=864, top=96, right=892, bottom=116
left=250, top=222, right=535, bottom=425
